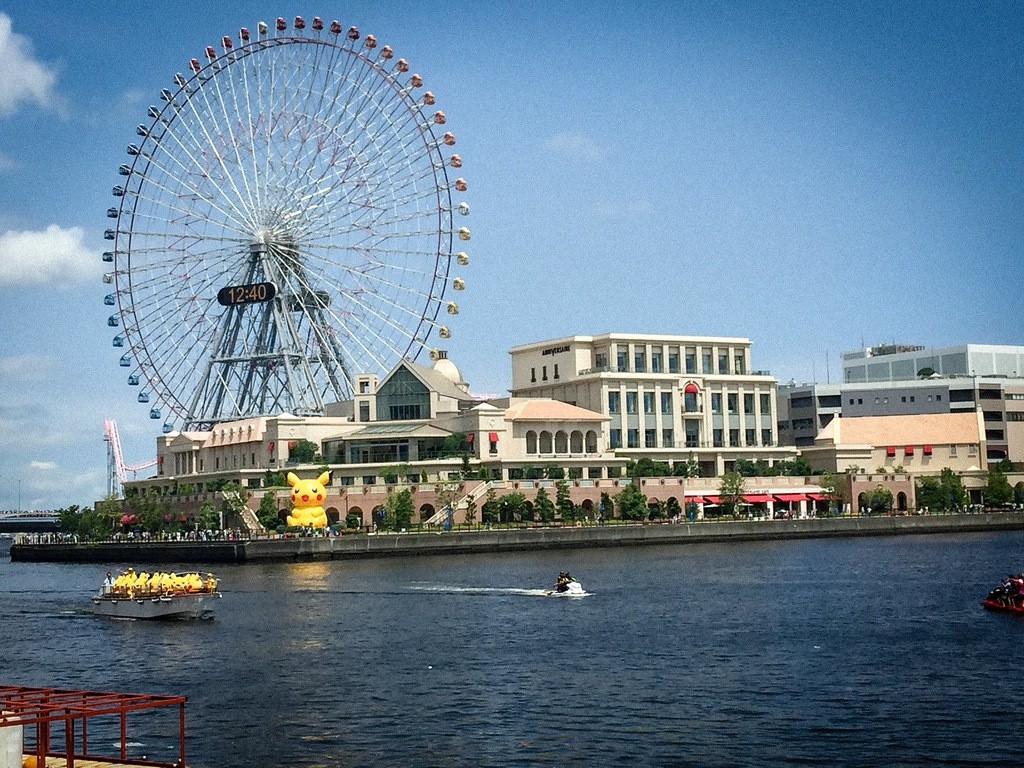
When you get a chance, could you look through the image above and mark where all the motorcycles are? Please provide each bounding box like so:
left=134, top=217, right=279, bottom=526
left=543, top=582, right=597, bottom=597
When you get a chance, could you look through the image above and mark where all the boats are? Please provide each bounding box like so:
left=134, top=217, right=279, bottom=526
left=979, top=587, right=1024, bottom=612
left=91, top=571, right=223, bottom=621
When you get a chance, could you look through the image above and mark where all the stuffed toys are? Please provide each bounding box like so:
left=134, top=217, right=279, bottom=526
left=114, top=567, right=202, bottom=595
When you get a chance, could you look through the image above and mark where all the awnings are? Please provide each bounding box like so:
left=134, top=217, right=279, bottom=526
left=740, top=494, right=777, bottom=502
left=705, top=496, right=741, bottom=504
left=807, top=493, right=843, bottom=500
left=684, top=496, right=707, bottom=503
left=775, top=495, right=810, bottom=501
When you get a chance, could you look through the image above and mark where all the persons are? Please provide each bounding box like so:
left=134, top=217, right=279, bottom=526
left=103, top=572, right=115, bottom=594
left=557, top=571, right=576, bottom=592
left=165, top=526, right=240, bottom=541
left=299, top=526, right=342, bottom=537
left=207, top=572, right=214, bottom=592
left=23, top=532, right=77, bottom=544
left=1001, top=574, right=1024, bottom=607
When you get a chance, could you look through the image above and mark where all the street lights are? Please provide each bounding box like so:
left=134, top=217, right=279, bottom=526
left=19, top=479, right=20, bottom=512
